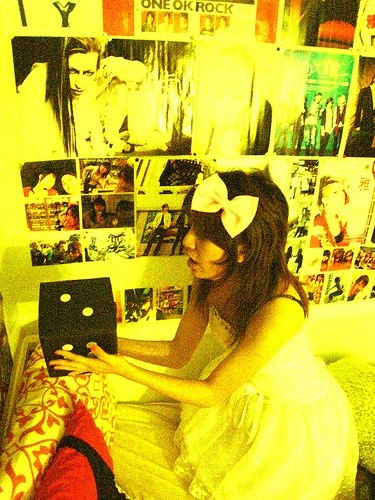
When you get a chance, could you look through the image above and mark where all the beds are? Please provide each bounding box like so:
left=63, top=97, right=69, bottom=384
left=0, top=299, right=375, bottom=500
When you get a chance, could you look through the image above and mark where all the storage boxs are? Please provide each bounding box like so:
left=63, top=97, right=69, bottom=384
left=37, top=276, right=119, bottom=377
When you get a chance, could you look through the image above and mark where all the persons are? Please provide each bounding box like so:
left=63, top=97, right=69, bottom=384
left=48, top=168, right=359, bottom=500
left=11, top=0, right=375, bottom=323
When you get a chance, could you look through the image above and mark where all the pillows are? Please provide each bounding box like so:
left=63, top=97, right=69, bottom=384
left=326, top=354, right=375, bottom=475
left=30, top=398, right=128, bottom=500
left=1, top=340, right=118, bottom=500
